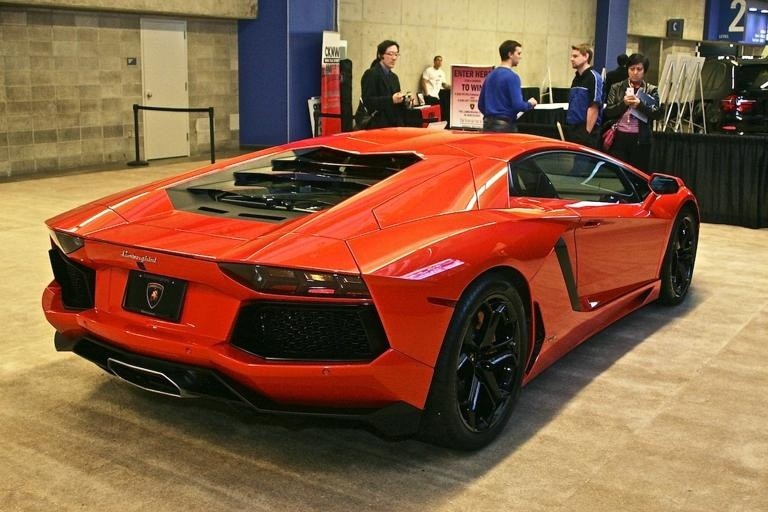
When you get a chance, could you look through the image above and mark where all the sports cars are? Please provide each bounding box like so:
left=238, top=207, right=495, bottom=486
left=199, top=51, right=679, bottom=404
left=42, top=124, right=703, bottom=456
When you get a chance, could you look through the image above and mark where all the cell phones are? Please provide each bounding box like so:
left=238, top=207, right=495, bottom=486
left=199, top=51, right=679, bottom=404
left=626, top=87, right=634, bottom=97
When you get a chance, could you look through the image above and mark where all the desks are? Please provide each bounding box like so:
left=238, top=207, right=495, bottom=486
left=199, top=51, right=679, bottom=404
left=631, top=128, right=766, bottom=233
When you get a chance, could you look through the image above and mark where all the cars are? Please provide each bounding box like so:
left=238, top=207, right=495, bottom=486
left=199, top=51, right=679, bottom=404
left=720, top=71, right=768, bottom=130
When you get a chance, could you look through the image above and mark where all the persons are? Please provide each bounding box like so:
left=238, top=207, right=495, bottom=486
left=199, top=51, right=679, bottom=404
left=605, top=54, right=659, bottom=176
left=602, top=55, right=629, bottom=104
left=569, top=44, right=602, bottom=150
left=361, top=40, right=416, bottom=127
left=421, top=56, right=451, bottom=105
left=478, top=41, right=537, bottom=133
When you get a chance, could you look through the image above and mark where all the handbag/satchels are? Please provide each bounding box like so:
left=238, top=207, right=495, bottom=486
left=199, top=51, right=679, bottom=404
left=354, top=98, right=373, bottom=131
left=602, top=124, right=619, bottom=149
